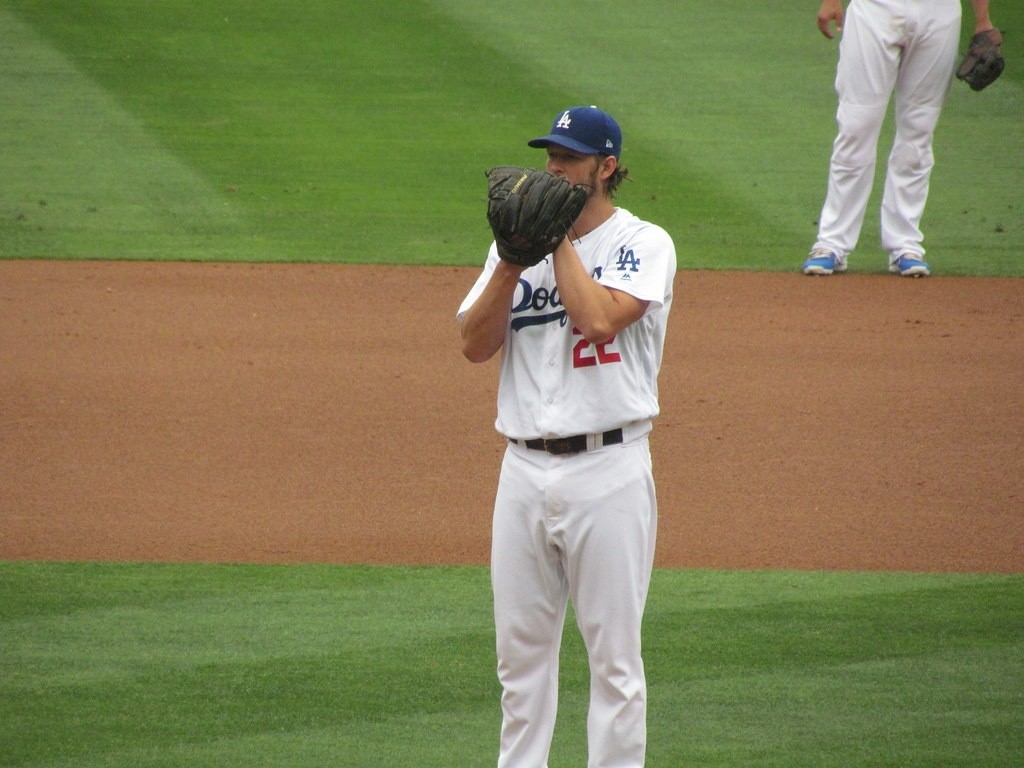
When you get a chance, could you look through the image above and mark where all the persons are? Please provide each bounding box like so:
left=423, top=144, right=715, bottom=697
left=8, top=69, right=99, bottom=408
left=800, top=0, right=1005, bottom=279
left=456, top=105, right=677, bottom=768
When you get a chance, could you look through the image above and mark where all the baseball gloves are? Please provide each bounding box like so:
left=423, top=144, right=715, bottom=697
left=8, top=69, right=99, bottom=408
left=484, top=166, right=589, bottom=268
left=954, top=28, right=1006, bottom=92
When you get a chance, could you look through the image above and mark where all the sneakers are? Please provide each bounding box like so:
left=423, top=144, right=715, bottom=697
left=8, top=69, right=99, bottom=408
left=803, top=247, right=848, bottom=274
left=889, top=253, right=930, bottom=278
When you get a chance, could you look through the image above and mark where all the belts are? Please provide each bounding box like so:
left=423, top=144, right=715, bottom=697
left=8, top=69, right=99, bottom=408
left=509, top=427, right=623, bottom=455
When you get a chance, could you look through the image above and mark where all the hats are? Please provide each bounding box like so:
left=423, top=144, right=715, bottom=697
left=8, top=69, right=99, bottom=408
left=527, top=105, right=622, bottom=159
